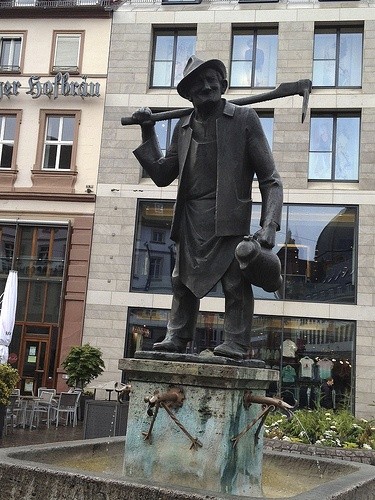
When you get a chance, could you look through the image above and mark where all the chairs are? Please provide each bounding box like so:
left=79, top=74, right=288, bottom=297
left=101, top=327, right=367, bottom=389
left=6, top=389, right=21, bottom=428
left=30, top=387, right=83, bottom=428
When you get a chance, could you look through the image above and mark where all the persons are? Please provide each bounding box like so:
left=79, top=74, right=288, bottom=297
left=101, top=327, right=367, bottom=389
left=319, top=123, right=331, bottom=151
left=325, top=36, right=349, bottom=85
left=245, top=39, right=267, bottom=84
left=134, top=56, right=284, bottom=359
left=319, top=377, right=337, bottom=412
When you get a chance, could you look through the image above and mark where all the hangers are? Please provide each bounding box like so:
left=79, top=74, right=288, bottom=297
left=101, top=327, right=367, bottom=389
left=303, top=355, right=310, bottom=360
left=286, top=365, right=292, bottom=369
left=322, top=357, right=330, bottom=361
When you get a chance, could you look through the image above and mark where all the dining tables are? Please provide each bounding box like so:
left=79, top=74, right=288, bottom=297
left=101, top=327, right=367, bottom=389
left=10, top=394, right=42, bottom=429
